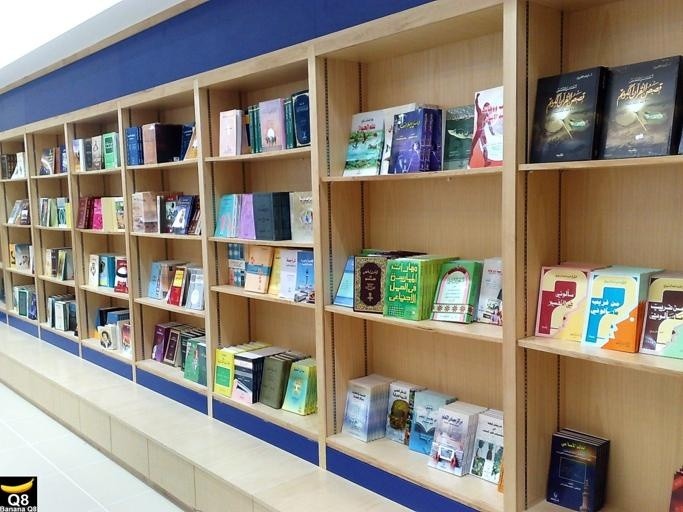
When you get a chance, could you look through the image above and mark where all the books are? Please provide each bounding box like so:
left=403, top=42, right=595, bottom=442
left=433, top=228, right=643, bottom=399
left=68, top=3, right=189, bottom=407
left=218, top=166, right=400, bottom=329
left=534, top=263, right=683, bottom=360
left=332, top=249, right=503, bottom=327
left=670, top=465, right=683, bottom=511
left=545, top=427, right=610, bottom=512
left=214, top=340, right=317, bottom=417
left=219, top=89, right=311, bottom=158
left=214, top=190, right=314, bottom=304
left=341, top=85, right=504, bottom=178
left=1, top=122, right=206, bottom=386
left=529, top=54, right=683, bottom=164
left=341, top=374, right=504, bottom=495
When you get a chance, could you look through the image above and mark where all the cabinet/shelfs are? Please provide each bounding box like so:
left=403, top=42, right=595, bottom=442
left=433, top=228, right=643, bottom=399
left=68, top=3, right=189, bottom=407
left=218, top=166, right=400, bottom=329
left=0, top=0, right=683, bottom=512
